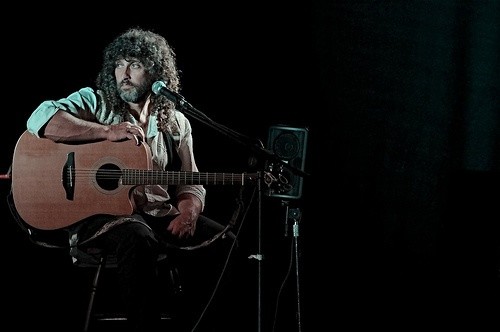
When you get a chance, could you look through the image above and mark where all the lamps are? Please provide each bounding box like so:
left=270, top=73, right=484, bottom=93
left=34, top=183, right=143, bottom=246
left=260, top=123, right=308, bottom=200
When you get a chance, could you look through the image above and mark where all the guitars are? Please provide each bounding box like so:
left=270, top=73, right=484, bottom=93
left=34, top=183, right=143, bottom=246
left=11, top=129, right=292, bottom=231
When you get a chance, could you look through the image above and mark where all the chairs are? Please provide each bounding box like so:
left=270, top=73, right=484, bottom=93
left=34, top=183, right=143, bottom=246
left=6, top=192, right=127, bottom=332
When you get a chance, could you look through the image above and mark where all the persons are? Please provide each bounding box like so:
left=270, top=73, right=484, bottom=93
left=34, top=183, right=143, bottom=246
left=27, top=30, right=237, bottom=332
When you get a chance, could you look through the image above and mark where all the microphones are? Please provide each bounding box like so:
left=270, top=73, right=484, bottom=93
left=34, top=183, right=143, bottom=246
left=151, top=81, right=193, bottom=110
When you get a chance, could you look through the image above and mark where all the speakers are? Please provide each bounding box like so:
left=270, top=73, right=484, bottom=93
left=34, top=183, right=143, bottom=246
left=262, top=126, right=307, bottom=198
